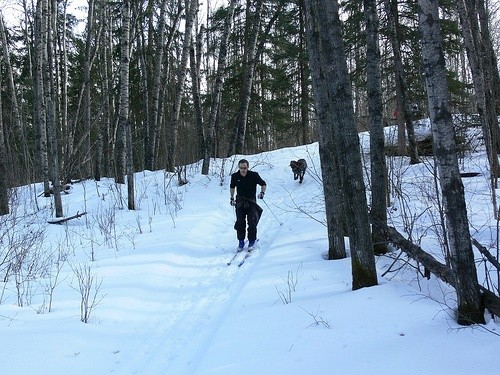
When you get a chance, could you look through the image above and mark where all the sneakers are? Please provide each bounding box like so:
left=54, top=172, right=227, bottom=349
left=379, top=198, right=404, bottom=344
left=237, top=240, right=244, bottom=252
left=247, top=240, right=256, bottom=251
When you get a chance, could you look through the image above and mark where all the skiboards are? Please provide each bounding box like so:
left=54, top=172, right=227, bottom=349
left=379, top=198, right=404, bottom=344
left=228, top=239, right=259, bottom=268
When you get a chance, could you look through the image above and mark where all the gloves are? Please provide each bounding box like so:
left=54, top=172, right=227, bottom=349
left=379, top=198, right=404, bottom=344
left=230, top=197, right=235, bottom=206
left=257, top=192, right=264, bottom=199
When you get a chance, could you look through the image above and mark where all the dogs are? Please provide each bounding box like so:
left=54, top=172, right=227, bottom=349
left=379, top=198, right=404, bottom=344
left=290, top=158, right=307, bottom=183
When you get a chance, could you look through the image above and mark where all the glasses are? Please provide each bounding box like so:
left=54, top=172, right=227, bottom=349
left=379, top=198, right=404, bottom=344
left=239, top=167, right=248, bottom=169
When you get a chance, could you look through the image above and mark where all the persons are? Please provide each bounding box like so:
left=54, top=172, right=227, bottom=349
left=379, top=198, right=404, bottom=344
left=229, top=159, right=266, bottom=251
